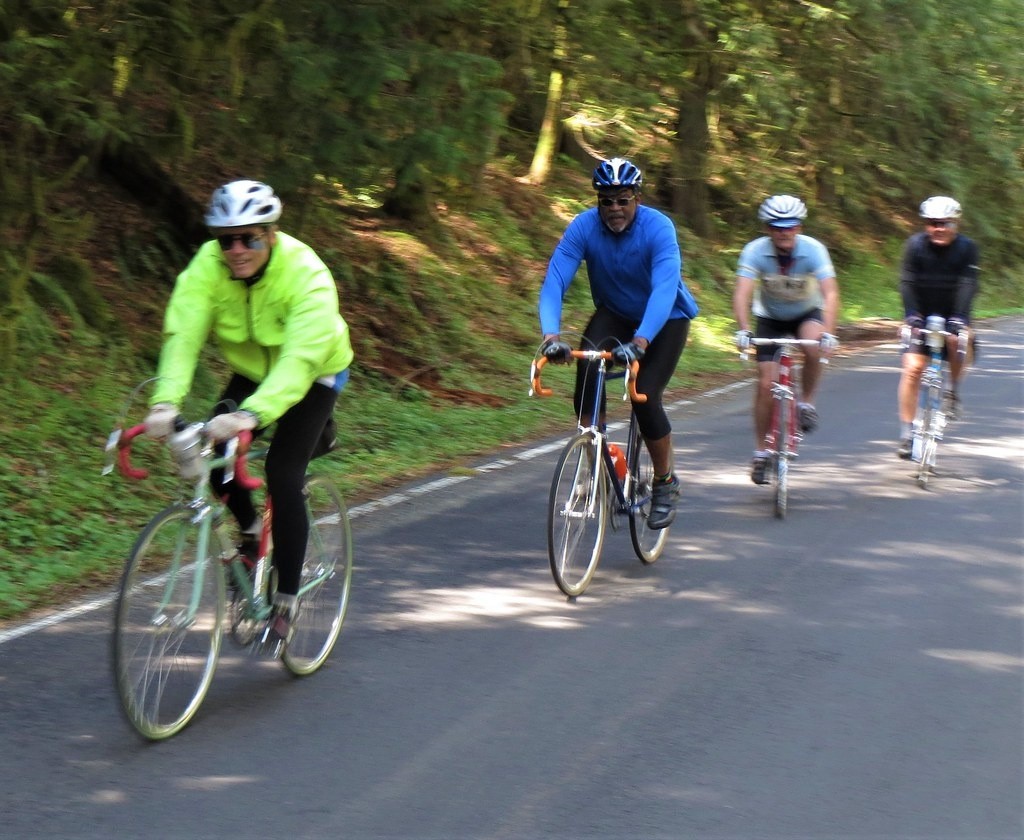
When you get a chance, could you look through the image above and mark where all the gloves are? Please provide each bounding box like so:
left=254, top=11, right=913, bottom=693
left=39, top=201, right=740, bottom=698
left=610, top=342, right=647, bottom=368
left=541, top=340, right=573, bottom=365
left=947, top=319, right=970, bottom=346
left=819, top=332, right=839, bottom=352
left=143, top=403, right=179, bottom=439
left=200, top=410, right=258, bottom=444
left=904, top=316, right=924, bottom=342
left=734, top=330, right=755, bottom=349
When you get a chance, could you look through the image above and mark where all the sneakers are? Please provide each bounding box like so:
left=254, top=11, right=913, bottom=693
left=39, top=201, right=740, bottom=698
left=752, top=455, right=772, bottom=483
left=264, top=594, right=297, bottom=636
left=796, top=404, right=818, bottom=434
left=646, top=474, right=682, bottom=530
left=226, top=526, right=260, bottom=589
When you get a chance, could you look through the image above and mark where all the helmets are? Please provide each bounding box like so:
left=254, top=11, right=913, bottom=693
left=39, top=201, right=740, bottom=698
left=758, top=194, right=808, bottom=228
left=919, top=196, right=963, bottom=219
left=203, top=179, right=284, bottom=230
left=592, top=157, right=642, bottom=197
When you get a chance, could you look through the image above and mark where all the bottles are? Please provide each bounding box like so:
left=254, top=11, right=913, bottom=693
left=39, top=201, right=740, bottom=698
left=608, top=445, right=627, bottom=479
left=165, top=415, right=206, bottom=480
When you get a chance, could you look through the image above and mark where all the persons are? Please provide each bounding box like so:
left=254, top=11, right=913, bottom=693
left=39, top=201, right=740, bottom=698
left=541, top=157, right=699, bottom=529
left=898, top=196, right=980, bottom=459
left=734, top=195, right=839, bottom=484
left=145, top=181, right=355, bottom=640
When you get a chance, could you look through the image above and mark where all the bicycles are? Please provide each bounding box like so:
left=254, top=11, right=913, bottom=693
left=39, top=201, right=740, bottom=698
left=731, top=333, right=838, bottom=521
left=903, top=310, right=977, bottom=491
left=100, top=374, right=353, bottom=745
left=527, top=329, right=679, bottom=597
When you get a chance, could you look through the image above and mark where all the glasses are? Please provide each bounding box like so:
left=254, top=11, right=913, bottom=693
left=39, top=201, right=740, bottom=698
left=926, top=220, right=957, bottom=227
left=219, top=232, right=267, bottom=251
left=770, top=227, right=804, bottom=232
left=597, top=198, right=635, bottom=205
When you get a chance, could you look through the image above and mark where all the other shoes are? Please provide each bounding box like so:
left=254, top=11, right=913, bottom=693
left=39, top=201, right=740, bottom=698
left=898, top=436, right=913, bottom=459
left=944, top=393, right=963, bottom=421
